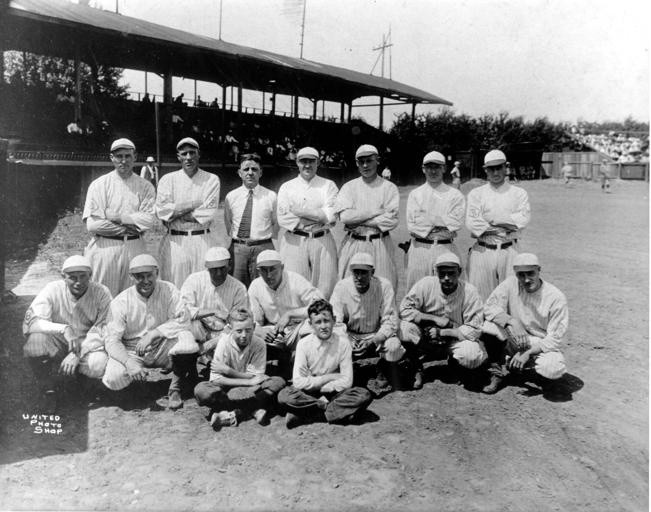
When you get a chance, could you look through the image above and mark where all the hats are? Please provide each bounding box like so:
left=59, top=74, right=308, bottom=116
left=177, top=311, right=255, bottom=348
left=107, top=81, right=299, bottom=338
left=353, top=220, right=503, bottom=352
left=109, top=137, right=507, bottom=169
left=61, top=245, right=542, bottom=276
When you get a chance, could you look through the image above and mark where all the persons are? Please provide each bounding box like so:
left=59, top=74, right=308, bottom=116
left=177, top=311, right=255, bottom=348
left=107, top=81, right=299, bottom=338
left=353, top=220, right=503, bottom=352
left=277, top=297, right=372, bottom=431
left=570, top=124, right=650, bottom=166
left=561, top=162, right=573, bottom=184
left=8, top=69, right=571, bottom=411
left=600, top=159, right=612, bottom=195
left=192, top=306, right=287, bottom=430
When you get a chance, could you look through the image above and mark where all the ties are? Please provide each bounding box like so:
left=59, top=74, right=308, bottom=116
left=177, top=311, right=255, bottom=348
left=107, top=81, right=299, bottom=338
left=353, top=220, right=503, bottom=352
left=236, top=190, right=252, bottom=238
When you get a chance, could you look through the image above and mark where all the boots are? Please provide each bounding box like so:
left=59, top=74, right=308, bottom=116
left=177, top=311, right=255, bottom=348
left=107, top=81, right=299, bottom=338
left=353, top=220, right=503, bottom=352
left=167, top=361, right=190, bottom=410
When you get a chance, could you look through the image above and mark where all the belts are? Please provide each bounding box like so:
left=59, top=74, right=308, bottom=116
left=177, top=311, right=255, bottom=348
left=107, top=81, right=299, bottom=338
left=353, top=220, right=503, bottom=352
left=97, top=230, right=516, bottom=249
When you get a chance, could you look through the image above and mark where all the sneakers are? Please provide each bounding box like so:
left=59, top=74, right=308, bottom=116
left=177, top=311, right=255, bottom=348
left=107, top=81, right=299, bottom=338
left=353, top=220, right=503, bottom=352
left=480, top=362, right=504, bottom=394
left=209, top=410, right=238, bottom=428
left=250, top=408, right=267, bottom=424
left=411, top=365, right=425, bottom=390
left=284, top=411, right=302, bottom=429
